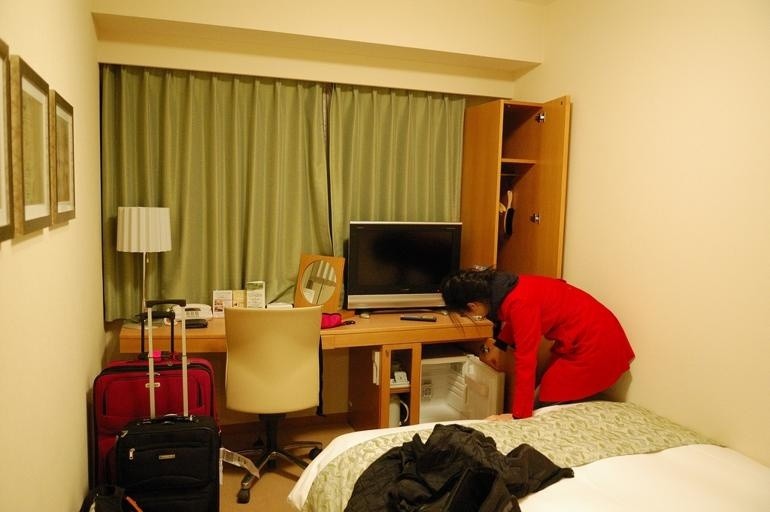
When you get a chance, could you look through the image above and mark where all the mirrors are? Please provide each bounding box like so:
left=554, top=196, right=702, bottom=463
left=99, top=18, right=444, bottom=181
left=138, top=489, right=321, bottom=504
left=294, top=254, right=346, bottom=314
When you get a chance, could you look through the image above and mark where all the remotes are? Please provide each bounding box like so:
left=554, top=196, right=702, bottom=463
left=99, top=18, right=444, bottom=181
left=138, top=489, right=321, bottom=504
left=400, top=313, right=437, bottom=322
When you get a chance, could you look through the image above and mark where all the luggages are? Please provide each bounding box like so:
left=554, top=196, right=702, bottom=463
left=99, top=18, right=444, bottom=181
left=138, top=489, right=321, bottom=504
left=116, top=299, right=219, bottom=512
left=93, top=311, right=215, bottom=491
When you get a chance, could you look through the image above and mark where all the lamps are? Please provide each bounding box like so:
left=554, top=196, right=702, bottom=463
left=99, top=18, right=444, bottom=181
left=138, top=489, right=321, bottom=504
left=117, top=207, right=172, bottom=330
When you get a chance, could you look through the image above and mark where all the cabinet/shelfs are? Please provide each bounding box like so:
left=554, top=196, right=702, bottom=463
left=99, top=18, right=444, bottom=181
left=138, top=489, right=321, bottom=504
left=461, top=95, right=573, bottom=375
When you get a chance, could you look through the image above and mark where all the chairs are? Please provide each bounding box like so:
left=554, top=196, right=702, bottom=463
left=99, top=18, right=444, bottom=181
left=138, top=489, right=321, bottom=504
left=224, top=306, right=322, bottom=504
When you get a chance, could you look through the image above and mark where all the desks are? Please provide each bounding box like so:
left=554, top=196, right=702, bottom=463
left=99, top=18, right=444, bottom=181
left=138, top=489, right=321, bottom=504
left=120, top=310, right=495, bottom=431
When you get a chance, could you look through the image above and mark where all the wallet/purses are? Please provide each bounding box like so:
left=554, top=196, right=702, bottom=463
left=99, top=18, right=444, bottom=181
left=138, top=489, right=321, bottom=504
left=185, top=319, right=208, bottom=328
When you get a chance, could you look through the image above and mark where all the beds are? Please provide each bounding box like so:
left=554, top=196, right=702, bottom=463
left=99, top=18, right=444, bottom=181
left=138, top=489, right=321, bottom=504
left=287, top=399, right=770, bottom=512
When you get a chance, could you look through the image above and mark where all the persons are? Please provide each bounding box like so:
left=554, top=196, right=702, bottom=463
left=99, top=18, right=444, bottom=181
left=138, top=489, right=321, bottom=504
left=441, top=265, right=636, bottom=423
left=214, top=300, right=224, bottom=311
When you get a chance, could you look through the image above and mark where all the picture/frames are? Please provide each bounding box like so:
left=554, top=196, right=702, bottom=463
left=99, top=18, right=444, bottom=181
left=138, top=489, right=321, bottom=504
left=0, top=38, right=75, bottom=243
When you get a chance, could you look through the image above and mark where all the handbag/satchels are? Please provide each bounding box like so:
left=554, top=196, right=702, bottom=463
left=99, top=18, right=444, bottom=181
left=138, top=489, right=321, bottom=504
left=321, top=313, right=341, bottom=329
left=95, top=484, right=123, bottom=512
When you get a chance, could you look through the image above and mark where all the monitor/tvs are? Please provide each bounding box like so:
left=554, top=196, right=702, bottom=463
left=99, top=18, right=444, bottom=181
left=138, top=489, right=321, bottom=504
left=347, top=218, right=464, bottom=319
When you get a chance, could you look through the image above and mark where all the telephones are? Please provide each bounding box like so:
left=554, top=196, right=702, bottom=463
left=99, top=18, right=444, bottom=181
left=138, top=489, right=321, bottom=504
left=172, top=303, right=213, bottom=320
